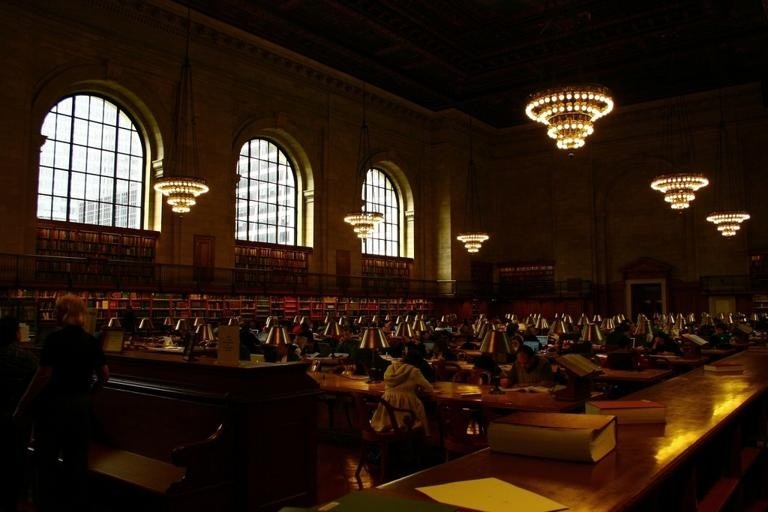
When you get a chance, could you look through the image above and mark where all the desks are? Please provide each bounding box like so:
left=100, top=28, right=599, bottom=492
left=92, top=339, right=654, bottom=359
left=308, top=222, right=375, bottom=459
left=3, top=316, right=768, bottom=510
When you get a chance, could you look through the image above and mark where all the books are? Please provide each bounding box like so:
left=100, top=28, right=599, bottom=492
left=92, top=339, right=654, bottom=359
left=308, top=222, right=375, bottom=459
left=486, top=409, right=618, bottom=462
left=584, top=399, right=665, bottom=424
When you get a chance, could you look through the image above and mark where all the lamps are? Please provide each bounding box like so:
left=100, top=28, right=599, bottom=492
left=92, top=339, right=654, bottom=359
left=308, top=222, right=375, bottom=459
left=152, top=0, right=209, bottom=214
left=342, top=59, right=382, bottom=243
left=456, top=77, right=490, bottom=254
left=650, top=0, right=709, bottom=211
left=522, top=0, right=616, bottom=151
left=706, top=0, right=751, bottom=237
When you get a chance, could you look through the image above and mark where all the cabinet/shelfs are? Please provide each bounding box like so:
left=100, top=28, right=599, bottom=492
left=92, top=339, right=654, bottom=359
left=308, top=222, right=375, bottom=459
left=496, top=260, right=556, bottom=293
left=2, top=228, right=434, bottom=322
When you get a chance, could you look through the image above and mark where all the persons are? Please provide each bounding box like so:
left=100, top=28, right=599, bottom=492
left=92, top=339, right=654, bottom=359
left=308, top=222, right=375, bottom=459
left=403, top=318, right=763, bottom=389
left=369, top=353, right=438, bottom=481
left=371, top=315, right=397, bottom=333
left=12, top=293, right=110, bottom=505
left=239, top=320, right=262, bottom=361
left=295, top=316, right=361, bottom=354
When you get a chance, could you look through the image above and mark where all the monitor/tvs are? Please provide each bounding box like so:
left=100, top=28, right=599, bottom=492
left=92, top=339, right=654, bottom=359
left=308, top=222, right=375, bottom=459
left=536, top=336, right=549, bottom=347
left=523, top=341, right=539, bottom=352
